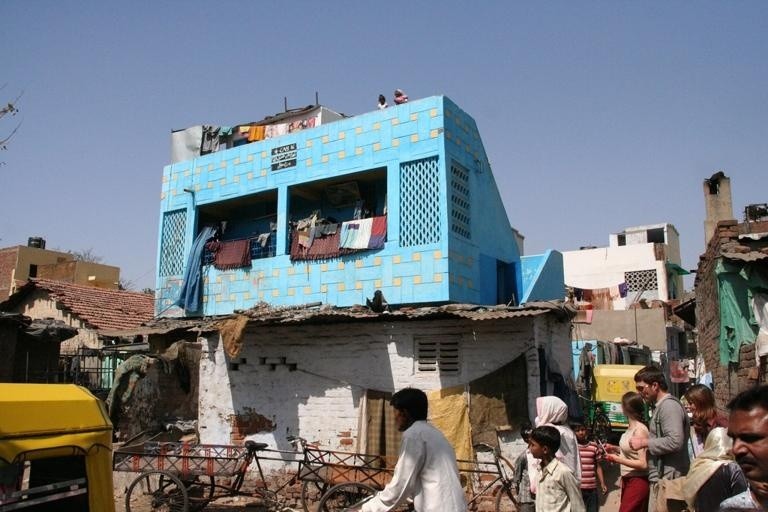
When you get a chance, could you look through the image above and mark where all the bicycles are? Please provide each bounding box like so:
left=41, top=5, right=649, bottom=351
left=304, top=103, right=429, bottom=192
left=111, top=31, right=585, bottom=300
left=576, top=393, right=612, bottom=446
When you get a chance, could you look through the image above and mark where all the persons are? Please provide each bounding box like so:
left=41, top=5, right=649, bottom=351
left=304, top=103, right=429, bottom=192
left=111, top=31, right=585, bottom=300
left=625, top=365, right=691, bottom=511
left=684, top=383, right=730, bottom=448
left=377, top=94, right=388, bottom=110
left=578, top=343, right=596, bottom=397
left=588, top=403, right=612, bottom=447
left=348, top=387, right=471, bottom=511
left=567, top=417, right=608, bottom=512
left=714, top=381, right=768, bottom=511
left=393, top=89, right=408, bottom=105
left=681, top=427, right=747, bottom=512
left=528, top=425, right=588, bottom=512
left=600, top=391, right=652, bottom=511
left=525, top=395, right=582, bottom=496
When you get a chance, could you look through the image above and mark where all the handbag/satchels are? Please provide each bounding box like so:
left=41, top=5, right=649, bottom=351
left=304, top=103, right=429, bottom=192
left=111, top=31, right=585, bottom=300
left=655, top=475, right=689, bottom=512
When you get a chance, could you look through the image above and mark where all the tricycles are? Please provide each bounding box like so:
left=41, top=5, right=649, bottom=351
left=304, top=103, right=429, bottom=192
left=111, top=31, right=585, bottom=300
left=297, top=441, right=521, bottom=512
left=112, top=438, right=348, bottom=512
